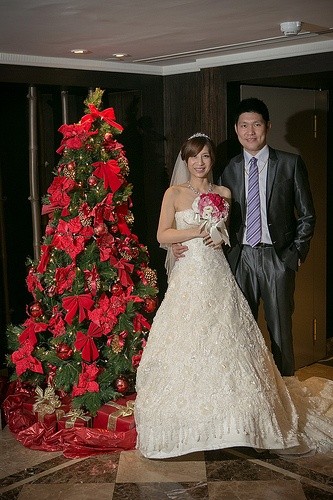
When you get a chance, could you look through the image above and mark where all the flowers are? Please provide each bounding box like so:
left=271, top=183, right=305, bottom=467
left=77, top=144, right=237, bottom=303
left=193, top=192, right=230, bottom=246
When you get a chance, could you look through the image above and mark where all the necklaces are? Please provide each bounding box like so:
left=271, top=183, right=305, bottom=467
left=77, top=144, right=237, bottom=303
left=186, top=180, right=212, bottom=196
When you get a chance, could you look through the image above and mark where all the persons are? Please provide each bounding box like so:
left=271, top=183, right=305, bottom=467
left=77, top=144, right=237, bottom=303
left=171, top=97, right=318, bottom=380
left=154, top=131, right=233, bottom=453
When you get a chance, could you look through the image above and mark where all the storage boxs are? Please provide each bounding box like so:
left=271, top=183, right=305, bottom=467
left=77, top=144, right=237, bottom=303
left=19, top=383, right=139, bottom=433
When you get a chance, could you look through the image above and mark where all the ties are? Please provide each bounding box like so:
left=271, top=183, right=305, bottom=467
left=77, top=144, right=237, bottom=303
left=245, top=156, right=261, bottom=247
